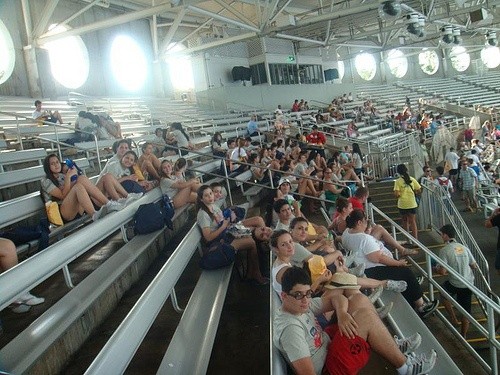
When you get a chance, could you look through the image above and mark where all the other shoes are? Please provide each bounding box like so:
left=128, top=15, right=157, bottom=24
left=368, top=283, right=383, bottom=304
left=92, top=191, right=143, bottom=222
left=412, top=244, right=419, bottom=248
left=404, top=243, right=413, bottom=245
left=245, top=274, right=269, bottom=286
left=9, top=301, right=31, bottom=313
left=376, top=301, right=393, bottom=319
left=347, top=263, right=365, bottom=276
left=13, top=291, right=44, bottom=306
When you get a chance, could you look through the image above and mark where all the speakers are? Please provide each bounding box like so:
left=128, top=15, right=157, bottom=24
left=324, top=68, right=338, bottom=81
left=232, top=66, right=252, bottom=80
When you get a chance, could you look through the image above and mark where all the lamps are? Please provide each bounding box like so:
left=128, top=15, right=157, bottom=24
left=380, top=0, right=401, bottom=17
left=405, top=14, right=426, bottom=38
left=486, top=30, right=499, bottom=48
left=440, top=26, right=462, bottom=45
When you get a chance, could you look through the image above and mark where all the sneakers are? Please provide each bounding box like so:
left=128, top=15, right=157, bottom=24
left=419, top=299, right=439, bottom=318
left=416, top=275, right=423, bottom=285
left=404, top=350, right=437, bottom=375
left=385, top=280, right=407, bottom=293
left=393, top=332, right=422, bottom=355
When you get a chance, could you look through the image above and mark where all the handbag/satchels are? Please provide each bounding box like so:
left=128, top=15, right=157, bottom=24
left=133, top=194, right=174, bottom=234
left=200, top=236, right=236, bottom=270
left=0, top=225, right=52, bottom=248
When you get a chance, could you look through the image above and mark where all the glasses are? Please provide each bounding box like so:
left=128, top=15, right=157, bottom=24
left=287, top=288, right=313, bottom=300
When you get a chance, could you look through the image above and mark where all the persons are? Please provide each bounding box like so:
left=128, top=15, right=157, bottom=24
left=393, top=164, right=423, bottom=246
left=32, top=93, right=500, bottom=375
left=436, top=224, right=479, bottom=342
left=0, top=238, right=45, bottom=314
left=274, top=267, right=438, bottom=375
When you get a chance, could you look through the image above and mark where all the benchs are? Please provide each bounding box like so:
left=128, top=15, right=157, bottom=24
left=0, top=73, right=500, bottom=375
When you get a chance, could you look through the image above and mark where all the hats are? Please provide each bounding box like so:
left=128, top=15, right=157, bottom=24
left=278, top=177, right=291, bottom=186
left=324, top=272, right=361, bottom=290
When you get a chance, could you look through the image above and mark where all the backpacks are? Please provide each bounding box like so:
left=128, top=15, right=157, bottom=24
left=438, top=177, right=450, bottom=197
